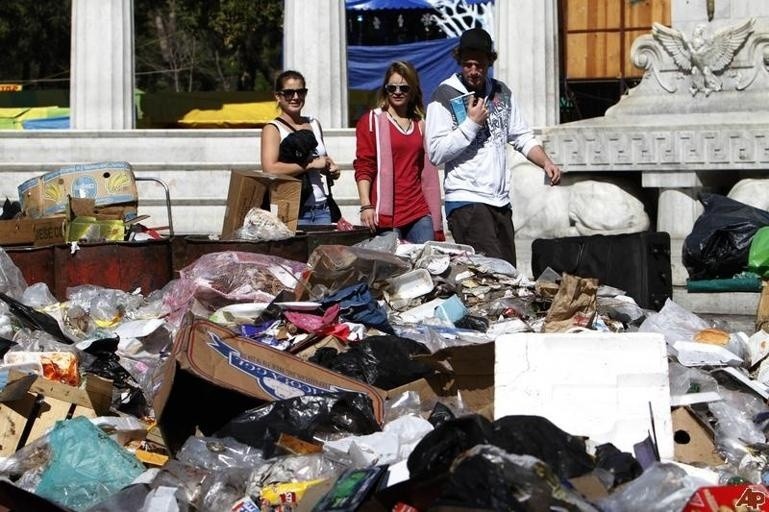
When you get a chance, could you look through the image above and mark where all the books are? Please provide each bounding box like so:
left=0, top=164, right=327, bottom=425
left=447, top=91, right=490, bottom=146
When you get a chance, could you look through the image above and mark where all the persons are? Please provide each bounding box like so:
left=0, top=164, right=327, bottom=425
left=352, top=60, right=445, bottom=244
left=423, top=26, right=564, bottom=269
left=261, top=69, right=342, bottom=225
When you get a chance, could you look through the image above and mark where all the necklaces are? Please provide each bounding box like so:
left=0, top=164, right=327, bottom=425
left=388, top=107, right=413, bottom=135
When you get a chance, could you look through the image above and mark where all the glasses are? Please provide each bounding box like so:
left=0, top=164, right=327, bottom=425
left=278, top=88, right=308, bottom=98
left=384, top=84, right=413, bottom=94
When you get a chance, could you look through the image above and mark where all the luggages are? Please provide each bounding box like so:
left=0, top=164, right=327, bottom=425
left=531, top=231, right=672, bottom=312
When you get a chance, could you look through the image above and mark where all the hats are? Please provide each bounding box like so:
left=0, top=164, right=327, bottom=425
left=453, top=29, right=497, bottom=61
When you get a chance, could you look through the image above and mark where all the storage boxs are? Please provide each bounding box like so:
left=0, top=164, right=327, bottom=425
left=0, top=161, right=150, bottom=246
left=221, top=167, right=303, bottom=238
left=151, top=311, right=388, bottom=465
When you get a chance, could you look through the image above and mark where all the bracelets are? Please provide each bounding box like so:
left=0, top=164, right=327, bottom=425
left=358, top=203, right=376, bottom=212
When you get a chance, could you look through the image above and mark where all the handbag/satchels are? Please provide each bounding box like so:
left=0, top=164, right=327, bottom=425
left=326, top=192, right=341, bottom=223
